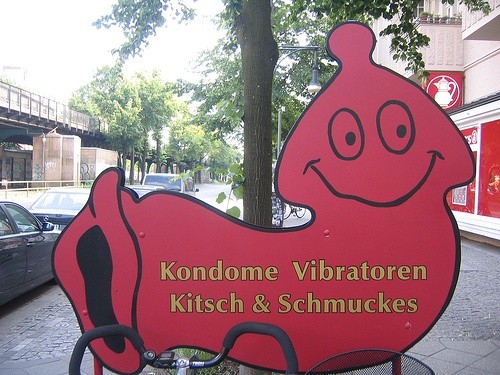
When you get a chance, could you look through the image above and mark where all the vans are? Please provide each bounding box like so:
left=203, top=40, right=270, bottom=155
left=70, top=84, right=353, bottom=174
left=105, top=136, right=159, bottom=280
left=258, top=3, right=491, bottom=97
left=143, top=172, right=200, bottom=199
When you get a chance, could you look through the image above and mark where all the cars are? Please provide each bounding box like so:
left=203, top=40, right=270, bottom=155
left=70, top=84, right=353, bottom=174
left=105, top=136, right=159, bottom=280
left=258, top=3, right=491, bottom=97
left=0, top=201, right=65, bottom=309
left=127, top=184, right=163, bottom=199
left=22, top=184, right=99, bottom=235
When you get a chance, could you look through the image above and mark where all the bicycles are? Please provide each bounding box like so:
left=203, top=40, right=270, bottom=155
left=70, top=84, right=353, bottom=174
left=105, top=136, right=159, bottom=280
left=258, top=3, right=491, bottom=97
left=276, top=200, right=307, bottom=220
